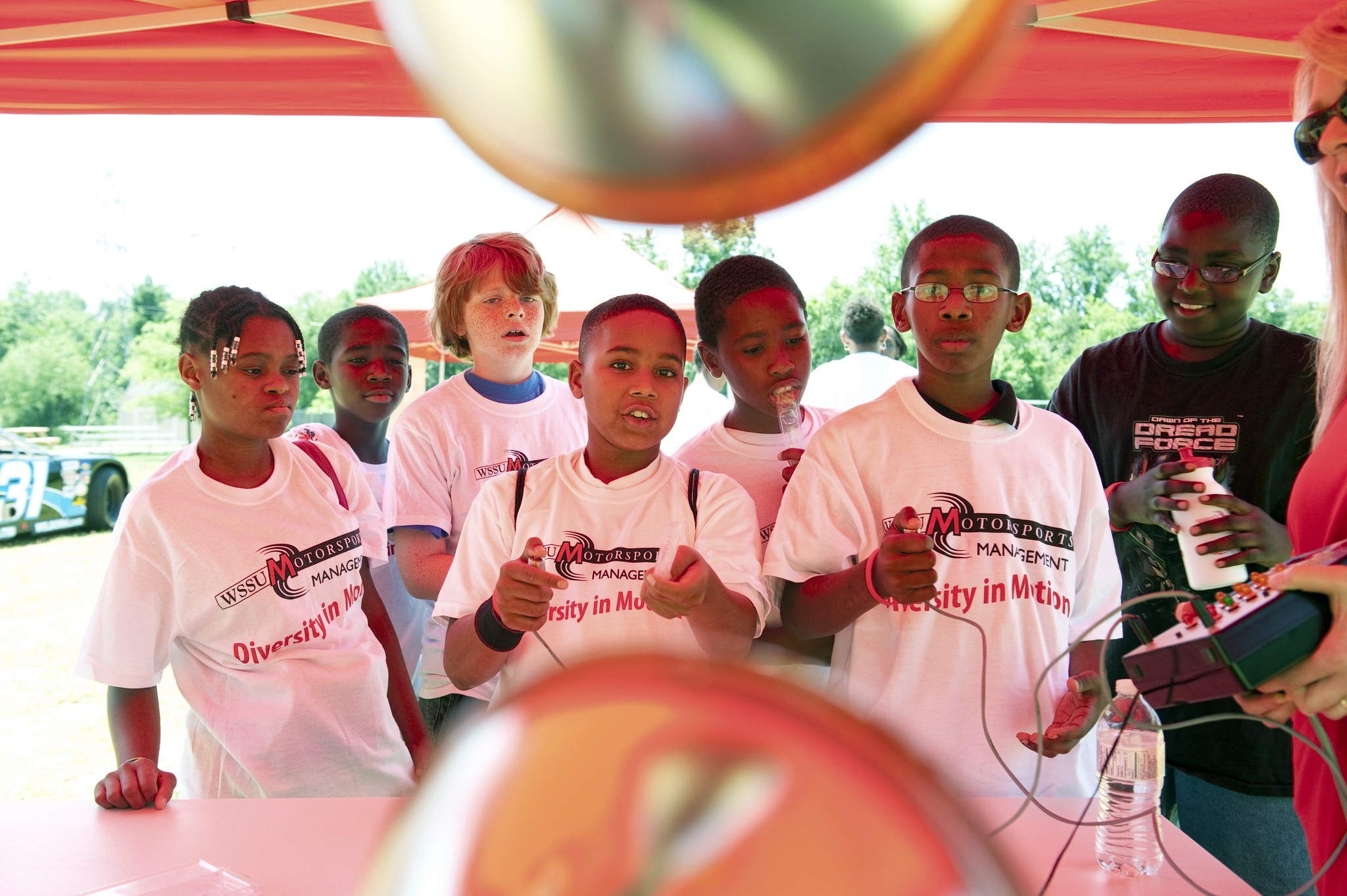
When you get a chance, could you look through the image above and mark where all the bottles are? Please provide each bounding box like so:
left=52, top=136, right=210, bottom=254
left=1094, top=678, right=1165, bottom=878
left=1167, top=445, right=1249, bottom=590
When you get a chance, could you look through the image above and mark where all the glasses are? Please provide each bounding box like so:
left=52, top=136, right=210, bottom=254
left=1151, top=247, right=1275, bottom=283
left=1294, top=91, right=1346, bottom=165
left=900, top=282, right=1019, bottom=303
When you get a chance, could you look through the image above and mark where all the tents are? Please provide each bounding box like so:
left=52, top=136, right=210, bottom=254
left=1, top=1, right=1347, bottom=123
left=352, top=206, right=700, bottom=384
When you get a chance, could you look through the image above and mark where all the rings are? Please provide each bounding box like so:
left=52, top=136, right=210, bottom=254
left=1340, top=699, right=1347, bottom=710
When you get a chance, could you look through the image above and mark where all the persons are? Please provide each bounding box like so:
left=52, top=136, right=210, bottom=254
left=800, top=304, right=918, bottom=413
left=1048, top=174, right=1317, bottom=896
left=282, top=305, right=434, bottom=679
left=670, top=255, right=843, bottom=694
left=1236, top=2, right=1347, bottom=896
left=881, top=324, right=907, bottom=361
left=431, top=294, right=771, bottom=711
left=382, top=231, right=586, bottom=754
left=73, top=285, right=430, bottom=810
left=659, top=344, right=733, bottom=454
left=760, top=215, right=1125, bottom=797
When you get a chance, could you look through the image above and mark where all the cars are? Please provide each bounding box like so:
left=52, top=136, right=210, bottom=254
left=0, top=428, right=130, bottom=542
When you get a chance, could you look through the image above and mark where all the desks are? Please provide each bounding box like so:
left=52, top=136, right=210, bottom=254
left=0, top=794, right=1263, bottom=896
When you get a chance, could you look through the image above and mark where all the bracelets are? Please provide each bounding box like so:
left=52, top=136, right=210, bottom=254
left=1105, top=482, right=1133, bottom=532
left=126, top=757, right=145, bottom=762
left=493, top=588, right=501, bottom=617
left=474, top=595, right=526, bottom=652
left=866, top=548, right=894, bottom=605
